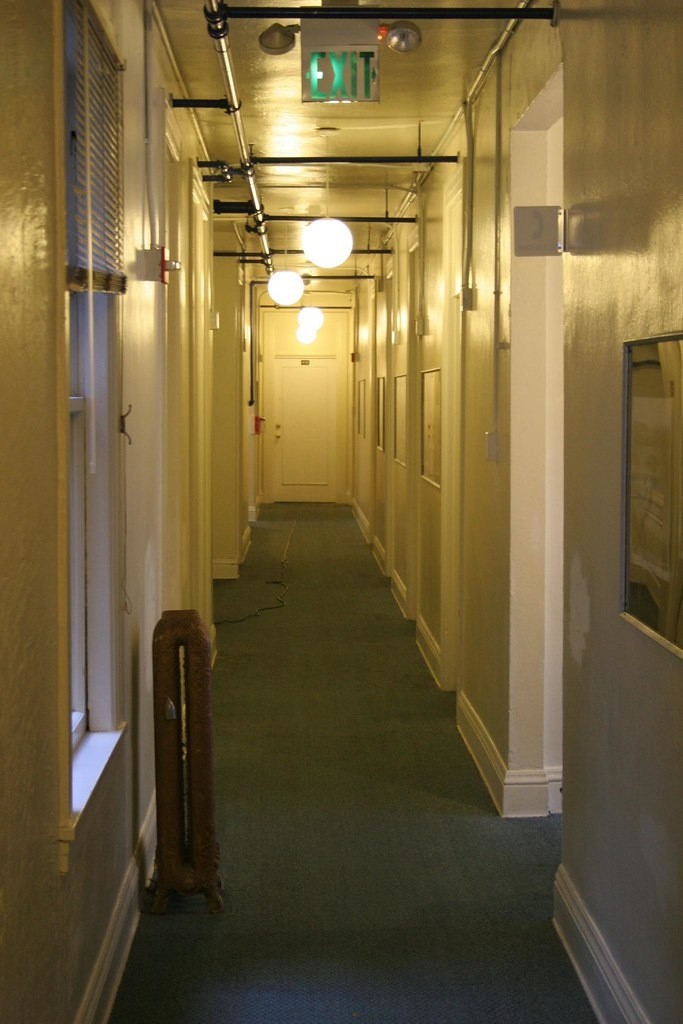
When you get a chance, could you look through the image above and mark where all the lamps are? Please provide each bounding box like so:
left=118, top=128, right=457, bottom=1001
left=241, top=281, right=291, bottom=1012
left=297, top=125, right=360, bottom=269
left=261, top=272, right=306, bottom=302
left=258, top=19, right=429, bottom=56
left=296, top=307, right=323, bottom=344
